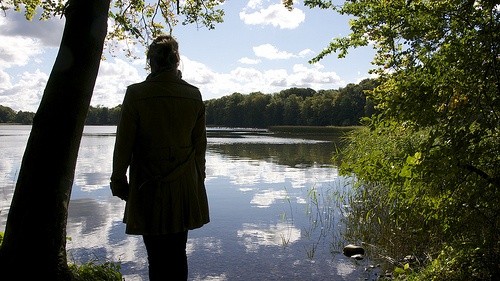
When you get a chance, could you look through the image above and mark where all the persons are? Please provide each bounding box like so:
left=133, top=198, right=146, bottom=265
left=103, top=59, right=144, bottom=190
left=111, top=35, right=210, bottom=281
